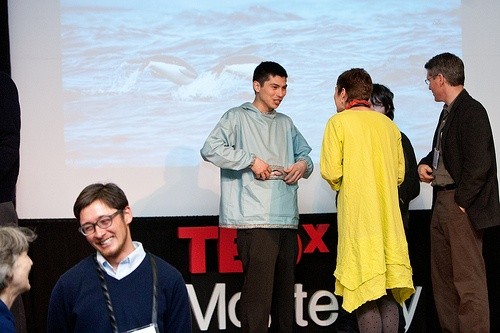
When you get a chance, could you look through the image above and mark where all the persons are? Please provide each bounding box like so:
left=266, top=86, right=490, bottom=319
left=319, top=66, right=416, bottom=332
left=199, top=60, right=314, bottom=332
left=1, top=71, right=26, bottom=333
left=417, top=51, right=499, bottom=333
left=365, top=83, right=421, bottom=240
left=1, top=224, right=37, bottom=332
left=46, top=182, right=196, bottom=332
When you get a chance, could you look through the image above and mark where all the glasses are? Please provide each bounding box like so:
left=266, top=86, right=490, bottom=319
left=425, top=74, right=438, bottom=85
left=370, top=103, right=391, bottom=108
left=79, top=209, right=123, bottom=236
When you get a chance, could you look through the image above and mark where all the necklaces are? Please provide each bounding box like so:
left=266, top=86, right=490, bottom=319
left=344, top=97, right=372, bottom=109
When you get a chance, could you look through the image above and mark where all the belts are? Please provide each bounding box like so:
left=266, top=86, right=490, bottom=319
left=433, top=183, right=458, bottom=192
